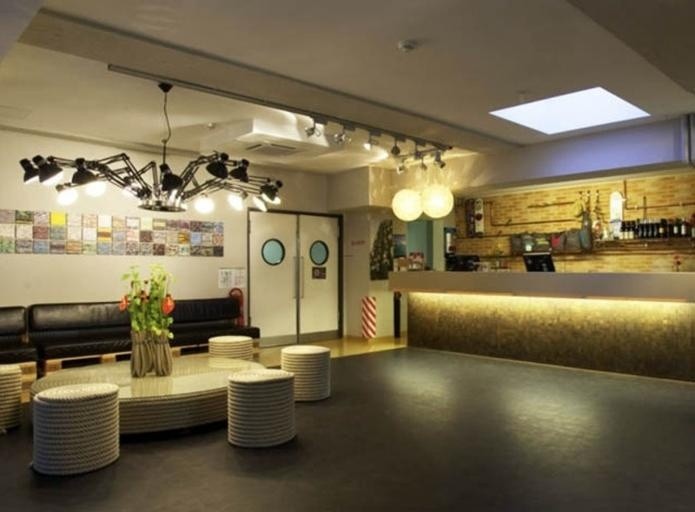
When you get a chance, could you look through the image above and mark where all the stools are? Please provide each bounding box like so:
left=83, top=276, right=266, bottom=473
left=227, top=369, right=298, bottom=448
left=32, top=382, right=120, bottom=475
left=0, top=364, right=23, bottom=434
left=207, top=335, right=254, bottom=365
left=280, top=344, right=331, bottom=401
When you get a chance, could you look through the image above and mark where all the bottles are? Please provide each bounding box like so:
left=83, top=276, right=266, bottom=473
left=393, top=250, right=424, bottom=272
left=619, top=217, right=695, bottom=240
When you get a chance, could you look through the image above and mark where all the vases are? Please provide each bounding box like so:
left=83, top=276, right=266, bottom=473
left=150, top=333, right=174, bottom=377
left=131, top=329, right=153, bottom=378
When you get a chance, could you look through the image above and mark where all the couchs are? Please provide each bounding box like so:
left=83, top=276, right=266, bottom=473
left=0, top=297, right=260, bottom=424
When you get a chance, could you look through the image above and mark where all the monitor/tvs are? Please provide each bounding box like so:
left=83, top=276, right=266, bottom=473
left=521, top=252, right=555, bottom=273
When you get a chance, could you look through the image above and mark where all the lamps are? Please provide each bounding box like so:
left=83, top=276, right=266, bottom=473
left=304, top=112, right=454, bottom=222
left=19, top=82, right=283, bottom=212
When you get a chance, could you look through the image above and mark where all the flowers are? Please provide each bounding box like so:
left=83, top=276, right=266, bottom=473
left=142, top=265, right=175, bottom=340
left=118, top=264, right=146, bottom=331
